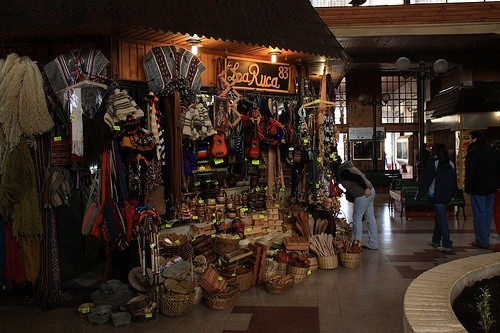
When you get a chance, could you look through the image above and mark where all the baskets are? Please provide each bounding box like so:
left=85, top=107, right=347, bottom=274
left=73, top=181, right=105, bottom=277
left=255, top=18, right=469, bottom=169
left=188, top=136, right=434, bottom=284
left=318, top=255, right=339, bottom=270
left=286, top=265, right=308, bottom=284
left=204, top=287, right=240, bottom=310
left=339, top=252, right=359, bottom=268
left=160, top=291, right=195, bottom=317
left=265, top=281, right=293, bottom=293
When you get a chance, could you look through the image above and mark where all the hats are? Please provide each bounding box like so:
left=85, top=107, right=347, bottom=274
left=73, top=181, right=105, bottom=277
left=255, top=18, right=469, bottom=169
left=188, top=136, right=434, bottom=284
left=178, top=100, right=214, bottom=140
left=103, top=88, right=159, bottom=192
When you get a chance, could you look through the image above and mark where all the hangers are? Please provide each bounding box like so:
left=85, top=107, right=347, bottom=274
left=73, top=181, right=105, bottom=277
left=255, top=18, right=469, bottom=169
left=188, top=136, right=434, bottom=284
left=61, top=73, right=107, bottom=91
left=302, top=96, right=336, bottom=108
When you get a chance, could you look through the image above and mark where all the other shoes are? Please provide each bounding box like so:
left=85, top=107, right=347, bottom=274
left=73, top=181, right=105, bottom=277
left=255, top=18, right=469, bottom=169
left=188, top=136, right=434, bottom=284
left=472, top=241, right=488, bottom=250
left=361, top=245, right=369, bottom=249
left=429, top=242, right=440, bottom=248
left=437, top=245, right=452, bottom=253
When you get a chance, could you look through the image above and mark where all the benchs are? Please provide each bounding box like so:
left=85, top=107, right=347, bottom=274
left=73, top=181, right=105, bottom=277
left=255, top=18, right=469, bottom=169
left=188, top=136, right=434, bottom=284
left=389, top=177, right=467, bottom=221
left=365, top=170, right=402, bottom=191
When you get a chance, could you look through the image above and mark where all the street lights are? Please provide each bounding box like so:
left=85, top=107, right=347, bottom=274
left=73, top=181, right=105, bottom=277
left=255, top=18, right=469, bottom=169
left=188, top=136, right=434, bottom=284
left=359, top=92, right=390, bottom=173
left=396, top=56, right=448, bottom=182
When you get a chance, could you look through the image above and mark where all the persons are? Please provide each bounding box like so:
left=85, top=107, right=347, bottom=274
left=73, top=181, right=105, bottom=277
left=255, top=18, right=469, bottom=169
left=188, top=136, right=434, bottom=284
left=414, top=143, right=458, bottom=252
left=331, top=159, right=379, bottom=250
left=464, top=131, right=500, bottom=249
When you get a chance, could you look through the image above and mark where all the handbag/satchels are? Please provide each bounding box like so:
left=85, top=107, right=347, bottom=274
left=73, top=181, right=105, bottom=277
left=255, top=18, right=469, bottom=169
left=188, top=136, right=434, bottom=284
left=81, top=194, right=137, bottom=250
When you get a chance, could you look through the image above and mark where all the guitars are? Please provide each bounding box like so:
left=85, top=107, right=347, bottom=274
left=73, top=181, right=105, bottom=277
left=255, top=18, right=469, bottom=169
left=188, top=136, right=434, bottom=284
left=249, top=110, right=261, bottom=160
left=209, top=131, right=229, bottom=159
